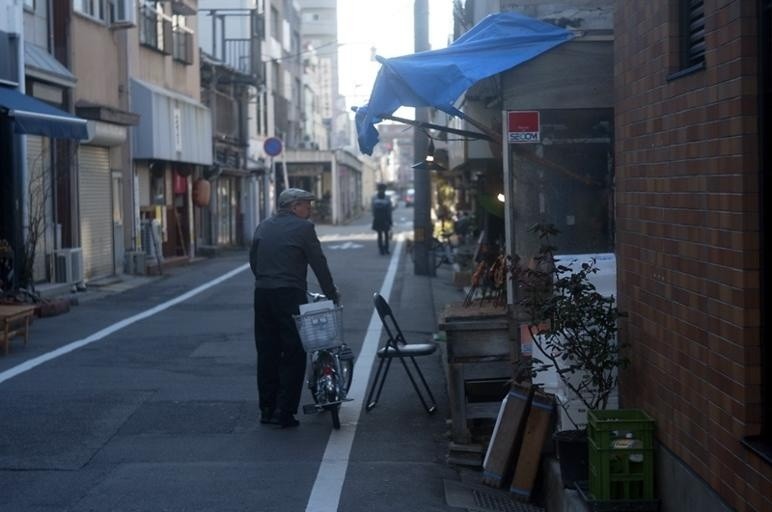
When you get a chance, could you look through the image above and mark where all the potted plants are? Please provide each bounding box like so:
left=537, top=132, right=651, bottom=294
left=502, top=221, right=633, bottom=490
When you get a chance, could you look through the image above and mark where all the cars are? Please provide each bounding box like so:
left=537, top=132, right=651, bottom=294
left=375, top=187, right=415, bottom=211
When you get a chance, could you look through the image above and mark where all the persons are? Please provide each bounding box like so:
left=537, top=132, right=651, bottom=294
left=249, top=188, right=338, bottom=430
left=371, top=184, right=392, bottom=255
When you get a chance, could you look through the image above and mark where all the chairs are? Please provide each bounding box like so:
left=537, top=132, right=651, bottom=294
left=366, top=292, right=438, bottom=415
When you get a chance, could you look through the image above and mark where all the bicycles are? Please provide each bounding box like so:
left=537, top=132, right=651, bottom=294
left=292, top=289, right=352, bottom=429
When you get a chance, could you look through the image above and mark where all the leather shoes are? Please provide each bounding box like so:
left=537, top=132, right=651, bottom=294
left=270, top=412, right=299, bottom=426
left=260, top=411, right=273, bottom=424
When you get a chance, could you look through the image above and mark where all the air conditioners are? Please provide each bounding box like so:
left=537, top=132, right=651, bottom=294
left=55, top=248, right=83, bottom=285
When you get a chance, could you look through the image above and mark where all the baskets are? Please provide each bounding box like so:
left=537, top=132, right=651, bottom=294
left=291, top=305, right=345, bottom=353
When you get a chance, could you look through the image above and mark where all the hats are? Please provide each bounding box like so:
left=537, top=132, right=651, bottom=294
left=278, top=188, right=316, bottom=206
